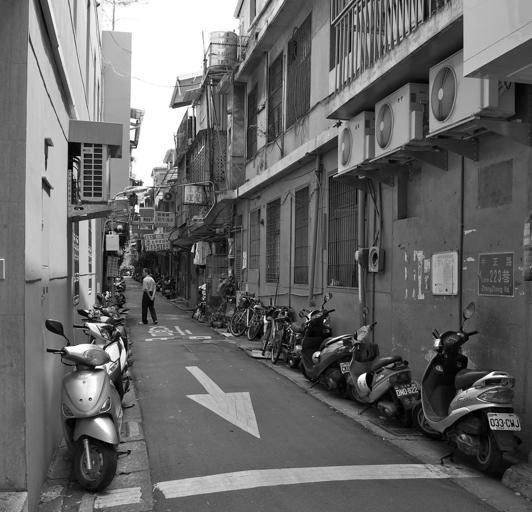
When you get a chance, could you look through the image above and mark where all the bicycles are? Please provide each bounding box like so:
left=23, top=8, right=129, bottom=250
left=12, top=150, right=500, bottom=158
left=229, top=292, right=305, bottom=368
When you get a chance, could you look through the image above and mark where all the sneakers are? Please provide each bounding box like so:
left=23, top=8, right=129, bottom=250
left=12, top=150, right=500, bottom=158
left=138, top=321, right=147, bottom=325
left=153, top=320, right=158, bottom=325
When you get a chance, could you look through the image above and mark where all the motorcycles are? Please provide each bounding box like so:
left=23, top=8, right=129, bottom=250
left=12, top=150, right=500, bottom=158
left=45, top=275, right=133, bottom=492
left=132, top=272, right=176, bottom=299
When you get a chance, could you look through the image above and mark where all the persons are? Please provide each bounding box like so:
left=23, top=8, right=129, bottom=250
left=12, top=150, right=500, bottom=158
left=137, top=268, right=159, bottom=325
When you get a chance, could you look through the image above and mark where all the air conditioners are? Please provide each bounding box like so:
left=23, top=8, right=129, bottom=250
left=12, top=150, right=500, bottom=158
left=337, top=111, right=387, bottom=177
left=375, top=83, right=440, bottom=160
left=429, top=48, right=515, bottom=140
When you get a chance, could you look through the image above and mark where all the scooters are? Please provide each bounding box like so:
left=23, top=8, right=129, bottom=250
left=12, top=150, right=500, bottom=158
left=298, top=292, right=354, bottom=400
left=410, top=301, right=522, bottom=475
left=346, top=306, right=421, bottom=429
